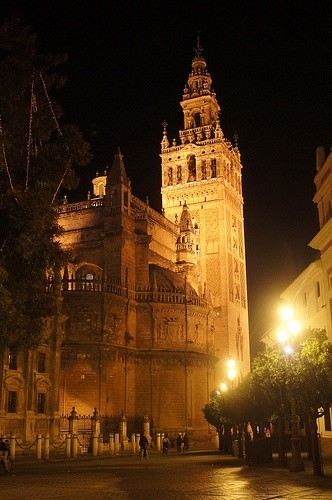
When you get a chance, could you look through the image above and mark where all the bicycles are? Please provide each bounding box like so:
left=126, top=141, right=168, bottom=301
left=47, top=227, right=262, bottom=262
left=162, top=443, right=170, bottom=455
left=176, top=442, right=189, bottom=455
left=138, top=444, right=149, bottom=461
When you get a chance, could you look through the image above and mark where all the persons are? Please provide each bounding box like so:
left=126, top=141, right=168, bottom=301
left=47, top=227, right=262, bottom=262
left=161, top=433, right=171, bottom=456
left=176, top=432, right=188, bottom=457
left=140, top=432, right=150, bottom=461
left=0, top=437, right=10, bottom=471
